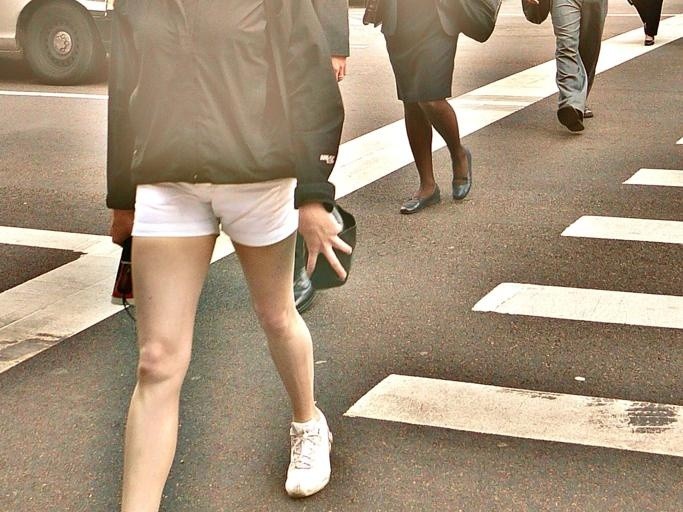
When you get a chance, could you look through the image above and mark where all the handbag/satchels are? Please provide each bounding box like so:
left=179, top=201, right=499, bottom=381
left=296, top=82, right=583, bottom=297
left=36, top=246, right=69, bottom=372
left=521, top=0, right=553, bottom=25
left=433, top=0, right=505, bottom=43
left=361, top=0, right=384, bottom=27
left=294, top=197, right=359, bottom=290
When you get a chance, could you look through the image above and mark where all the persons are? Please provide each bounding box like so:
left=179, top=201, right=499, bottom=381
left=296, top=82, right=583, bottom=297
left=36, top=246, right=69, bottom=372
left=521, top=0, right=609, bottom=132
left=293, top=0, right=350, bottom=313
left=360, top=0, right=502, bottom=214
left=106, top=0, right=344, bottom=512
left=627, top=0, right=663, bottom=46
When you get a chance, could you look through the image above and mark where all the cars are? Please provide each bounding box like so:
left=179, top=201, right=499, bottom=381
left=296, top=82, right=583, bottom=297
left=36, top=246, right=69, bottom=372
left=0, top=0, right=115, bottom=89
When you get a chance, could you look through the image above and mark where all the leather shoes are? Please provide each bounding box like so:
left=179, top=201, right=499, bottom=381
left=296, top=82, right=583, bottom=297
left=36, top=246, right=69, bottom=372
left=450, top=148, right=472, bottom=201
left=399, top=183, right=441, bottom=215
left=643, top=22, right=656, bottom=47
left=556, top=104, right=585, bottom=132
left=584, top=106, right=593, bottom=119
left=292, top=266, right=317, bottom=313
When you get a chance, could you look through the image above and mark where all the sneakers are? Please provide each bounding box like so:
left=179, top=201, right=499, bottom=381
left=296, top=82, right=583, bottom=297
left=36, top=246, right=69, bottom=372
left=283, top=400, right=333, bottom=498
